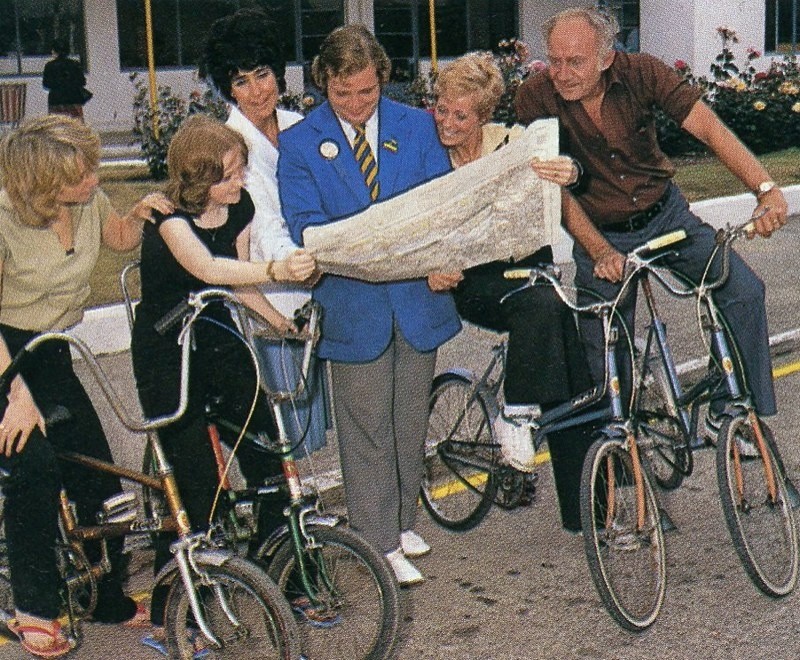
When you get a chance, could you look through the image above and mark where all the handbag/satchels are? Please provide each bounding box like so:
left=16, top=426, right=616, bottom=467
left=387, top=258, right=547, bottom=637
left=80, top=88, right=93, bottom=106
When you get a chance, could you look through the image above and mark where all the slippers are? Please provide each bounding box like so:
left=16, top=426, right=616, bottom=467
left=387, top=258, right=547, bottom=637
left=141, top=631, right=210, bottom=660
left=7, top=618, right=72, bottom=657
left=122, top=604, right=153, bottom=628
left=291, top=600, right=342, bottom=628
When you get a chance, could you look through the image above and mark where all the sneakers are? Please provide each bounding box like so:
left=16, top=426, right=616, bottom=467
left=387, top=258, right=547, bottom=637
left=599, top=522, right=641, bottom=552
left=703, top=407, right=763, bottom=459
left=493, top=406, right=539, bottom=473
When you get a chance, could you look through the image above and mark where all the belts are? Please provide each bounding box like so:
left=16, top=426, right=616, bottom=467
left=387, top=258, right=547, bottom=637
left=597, top=178, right=672, bottom=234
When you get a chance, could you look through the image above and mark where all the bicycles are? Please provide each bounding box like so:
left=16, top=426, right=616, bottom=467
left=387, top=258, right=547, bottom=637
left=0, top=257, right=402, bottom=659
left=419, top=203, right=799, bottom=633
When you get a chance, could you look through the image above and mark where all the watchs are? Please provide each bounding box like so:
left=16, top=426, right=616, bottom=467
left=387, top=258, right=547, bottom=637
left=755, top=181, right=776, bottom=196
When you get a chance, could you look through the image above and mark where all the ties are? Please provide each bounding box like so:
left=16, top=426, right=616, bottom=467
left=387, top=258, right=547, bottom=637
left=351, top=124, right=380, bottom=202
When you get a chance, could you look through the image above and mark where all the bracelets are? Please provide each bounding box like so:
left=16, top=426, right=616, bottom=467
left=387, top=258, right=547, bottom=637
left=266, top=260, right=276, bottom=283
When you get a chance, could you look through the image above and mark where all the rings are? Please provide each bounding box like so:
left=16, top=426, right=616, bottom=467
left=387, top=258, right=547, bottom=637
left=772, top=219, right=778, bottom=225
left=0, top=424, right=8, bottom=433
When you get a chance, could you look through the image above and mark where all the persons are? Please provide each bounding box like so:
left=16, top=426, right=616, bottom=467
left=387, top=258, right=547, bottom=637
left=514, top=7, right=788, bottom=460
left=435, top=49, right=644, bottom=553
left=42, top=39, right=93, bottom=124
left=132, top=114, right=345, bottom=658
left=0, top=114, right=175, bottom=658
left=200, top=8, right=333, bottom=458
left=278, top=23, right=465, bottom=588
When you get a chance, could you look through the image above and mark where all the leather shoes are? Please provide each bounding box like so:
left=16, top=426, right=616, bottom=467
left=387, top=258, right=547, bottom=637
left=357, top=548, right=425, bottom=588
left=401, top=528, right=431, bottom=558
